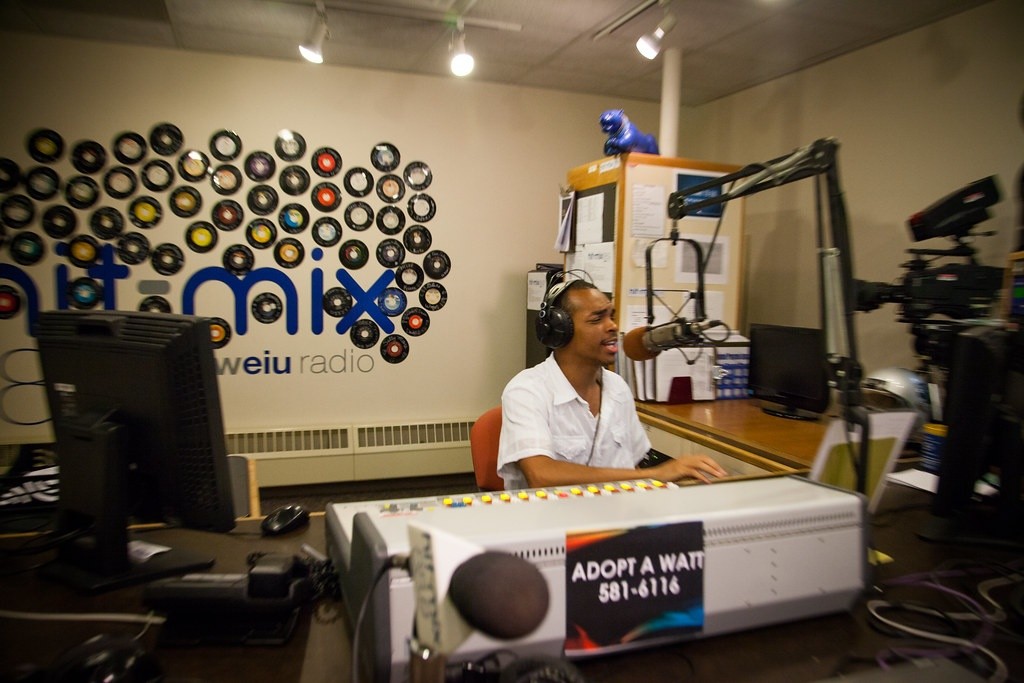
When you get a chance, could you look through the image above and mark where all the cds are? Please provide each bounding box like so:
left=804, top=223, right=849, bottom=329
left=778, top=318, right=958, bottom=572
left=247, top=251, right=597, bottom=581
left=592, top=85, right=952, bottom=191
left=0, top=121, right=452, bottom=364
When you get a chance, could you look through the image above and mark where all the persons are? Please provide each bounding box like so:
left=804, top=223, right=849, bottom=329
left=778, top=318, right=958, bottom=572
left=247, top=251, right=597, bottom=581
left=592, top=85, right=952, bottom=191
left=497, top=280, right=728, bottom=491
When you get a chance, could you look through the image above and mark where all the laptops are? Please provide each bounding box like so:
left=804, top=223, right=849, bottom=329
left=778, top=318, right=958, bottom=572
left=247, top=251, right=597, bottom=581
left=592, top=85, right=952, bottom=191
left=809, top=410, right=918, bottom=515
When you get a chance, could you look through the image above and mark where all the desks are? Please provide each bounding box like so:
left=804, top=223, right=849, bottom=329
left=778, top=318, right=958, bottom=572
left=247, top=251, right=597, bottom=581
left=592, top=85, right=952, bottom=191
left=0, top=402, right=1024, bottom=683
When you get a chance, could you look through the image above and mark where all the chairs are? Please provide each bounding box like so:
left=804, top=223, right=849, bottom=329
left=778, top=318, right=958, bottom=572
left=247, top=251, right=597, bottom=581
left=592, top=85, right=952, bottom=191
left=472, top=403, right=501, bottom=490
left=228, top=454, right=259, bottom=519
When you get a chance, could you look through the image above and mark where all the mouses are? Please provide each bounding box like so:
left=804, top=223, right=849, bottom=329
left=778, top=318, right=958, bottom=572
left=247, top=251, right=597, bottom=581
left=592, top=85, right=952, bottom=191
left=259, top=504, right=310, bottom=535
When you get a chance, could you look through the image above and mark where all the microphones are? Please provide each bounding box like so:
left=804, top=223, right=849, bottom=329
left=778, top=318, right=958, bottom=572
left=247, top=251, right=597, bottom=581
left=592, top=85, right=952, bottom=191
left=623, top=318, right=721, bottom=361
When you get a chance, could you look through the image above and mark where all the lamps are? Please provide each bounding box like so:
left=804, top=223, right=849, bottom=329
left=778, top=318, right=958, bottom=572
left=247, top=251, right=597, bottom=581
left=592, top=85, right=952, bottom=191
left=300, top=0, right=329, bottom=63
left=448, top=23, right=474, bottom=77
left=639, top=1, right=675, bottom=58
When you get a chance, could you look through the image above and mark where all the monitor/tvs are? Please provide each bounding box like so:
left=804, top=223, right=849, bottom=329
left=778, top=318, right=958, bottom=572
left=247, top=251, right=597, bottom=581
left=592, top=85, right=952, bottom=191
left=749, top=323, right=830, bottom=421
left=32, top=306, right=237, bottom=589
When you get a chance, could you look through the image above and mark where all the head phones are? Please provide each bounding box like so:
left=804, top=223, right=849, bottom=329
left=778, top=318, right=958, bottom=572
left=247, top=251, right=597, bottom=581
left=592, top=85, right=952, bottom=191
left=535, top=269, right=595, bottom=349
